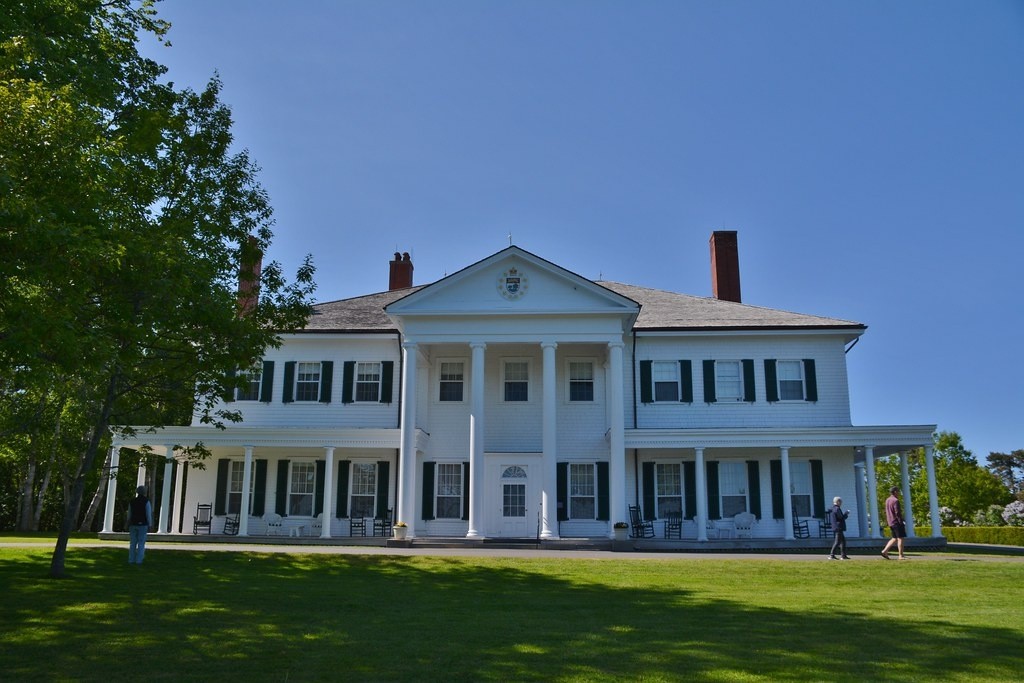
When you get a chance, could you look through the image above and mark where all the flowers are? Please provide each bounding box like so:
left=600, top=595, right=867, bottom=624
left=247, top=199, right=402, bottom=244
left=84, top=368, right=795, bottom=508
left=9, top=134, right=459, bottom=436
left=615, top=522, right=630, bottom=528
left=395, top=522, right=408, bottom=527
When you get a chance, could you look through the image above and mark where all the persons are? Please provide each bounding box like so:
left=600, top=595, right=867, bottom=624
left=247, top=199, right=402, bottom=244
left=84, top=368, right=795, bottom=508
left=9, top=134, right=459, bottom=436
left=881, top=487, right=911, bottom=560
left=828, top=497, right=850, bottom=560
left=126, top=485, right=152, bottom=566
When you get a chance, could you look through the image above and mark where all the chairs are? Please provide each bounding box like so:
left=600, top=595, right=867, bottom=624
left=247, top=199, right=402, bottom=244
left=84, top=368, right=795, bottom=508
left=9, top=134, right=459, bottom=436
left=819, top=508, right=836, bottom=540
left=350, top=511, right=367, bottom=537
left=223, top=513, right=241, bottom=535
left=629, top=504, right=656, bottom=539
left=193, top=501, right=213, bottom=535
left=310, top=513, right=324, bottom=536
left=693, top=516, right=716, bottom=540
left=664, top=511, right=683, bottom=539
left=732, top=513, right=755, bottom=539
left=792, top=508, right=810, bottom=539
left=262, top=513, right=282, bottom=535
left=372, top=507, right=393, bottom=537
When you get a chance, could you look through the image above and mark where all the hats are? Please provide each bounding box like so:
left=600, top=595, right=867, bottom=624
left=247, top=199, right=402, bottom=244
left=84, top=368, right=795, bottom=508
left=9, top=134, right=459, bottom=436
left=136, top=486, right=147, bottom=496
left=890, top=486, right=900, bottom=493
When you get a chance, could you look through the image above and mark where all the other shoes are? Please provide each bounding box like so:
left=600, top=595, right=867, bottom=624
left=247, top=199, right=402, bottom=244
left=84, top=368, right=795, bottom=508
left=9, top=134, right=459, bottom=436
left=840, top=556, right=851, bottom=560
left=829, top=555, right=837, bottom=560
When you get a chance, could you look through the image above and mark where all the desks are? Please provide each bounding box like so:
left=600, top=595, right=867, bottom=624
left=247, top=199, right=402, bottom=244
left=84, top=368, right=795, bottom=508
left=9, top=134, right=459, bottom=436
left=716, top=524, right=733, bottom=539
left=288, top=525, right=304, bottom=537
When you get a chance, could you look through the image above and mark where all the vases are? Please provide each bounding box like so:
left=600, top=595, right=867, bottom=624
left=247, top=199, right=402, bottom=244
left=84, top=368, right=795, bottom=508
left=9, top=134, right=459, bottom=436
left=393, top=526, right=408, bottom=539
left=615, top=526, right=629, bottom=542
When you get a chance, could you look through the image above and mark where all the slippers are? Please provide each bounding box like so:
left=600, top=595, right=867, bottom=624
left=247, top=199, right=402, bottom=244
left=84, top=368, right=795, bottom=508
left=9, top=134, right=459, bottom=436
left=881, top=552, right=890, bottom=560
left=899, top=557, right=910, bottom=561
